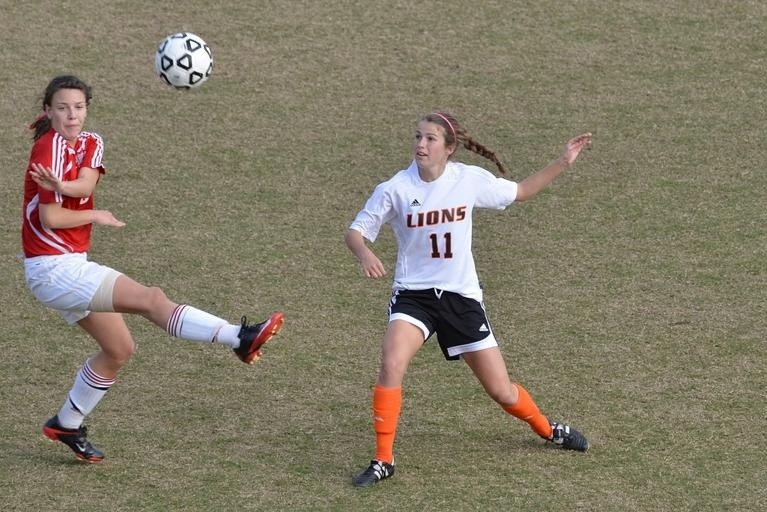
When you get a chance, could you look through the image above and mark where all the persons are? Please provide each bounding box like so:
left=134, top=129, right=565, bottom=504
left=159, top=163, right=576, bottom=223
left=21, top=76, right=284, bottom=464
left=344, top=112, right=592, bottom=487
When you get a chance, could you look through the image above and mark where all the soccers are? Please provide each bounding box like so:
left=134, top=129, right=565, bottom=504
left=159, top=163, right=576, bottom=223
left=154, top=33, right=213, bottom=89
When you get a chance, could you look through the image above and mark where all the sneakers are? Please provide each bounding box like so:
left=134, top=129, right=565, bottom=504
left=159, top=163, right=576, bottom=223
left=353, top=458, right=394, bottom=486
left=550, top=423, right=588, bottom=452
left=232, top=311, right=282, bottom=364
left=43, top=416, right=105, bottom=463
